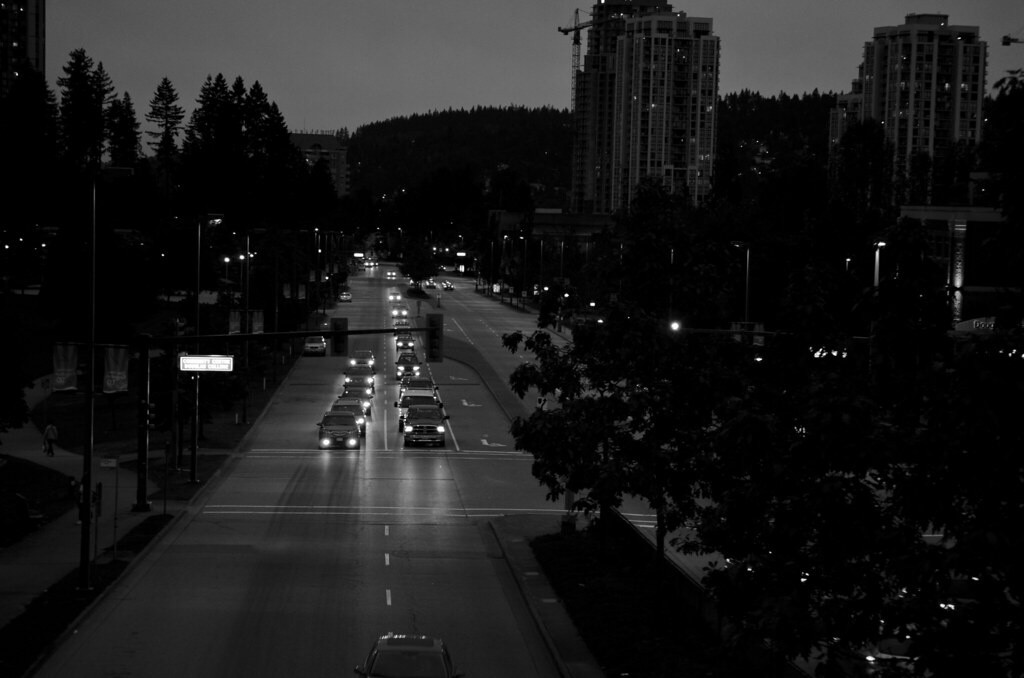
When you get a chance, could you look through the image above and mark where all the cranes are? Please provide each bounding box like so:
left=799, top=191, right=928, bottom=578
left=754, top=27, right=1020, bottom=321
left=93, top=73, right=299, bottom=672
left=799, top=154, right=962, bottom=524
left=557, top=8, right=594, bottom=109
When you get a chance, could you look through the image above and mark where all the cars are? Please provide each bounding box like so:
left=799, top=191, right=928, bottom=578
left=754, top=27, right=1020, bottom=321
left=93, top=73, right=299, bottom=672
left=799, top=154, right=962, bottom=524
left=394, top=353, right=422, bottom=380
left=427, top=279, right=436, bottom=289
left=316, top=350, right=376, bottom=449
left=394, top=376, right=444, bottom=432
left=338, top=292, right=352, bottom=303
left=304, top=335, right=326, bottom=356
left=401, top=404, right=450, bottom=447
left=387, top=269, right=397, bottom=277
left=354, top=633, right=464, bottom=678
left=442, top=280, right=454, bottom=290
left=388, top=293, right=416, bottom=352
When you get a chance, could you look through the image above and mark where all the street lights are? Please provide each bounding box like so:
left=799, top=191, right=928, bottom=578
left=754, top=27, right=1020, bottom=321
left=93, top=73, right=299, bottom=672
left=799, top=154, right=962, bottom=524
left=661, top=241, right=674, bottom=325
left=869, top=239, right=887, bottom=363
left=186, top=218, right=223, bottom=485
left=611, top=238, right=624, bottom=304
left=76, top=166, right=136, bottom=588
left=731, top=240, right=750, bottom=321
left=486, top=234, right=591, bottom=332
left=226, top=228, right=344, bottom=368
